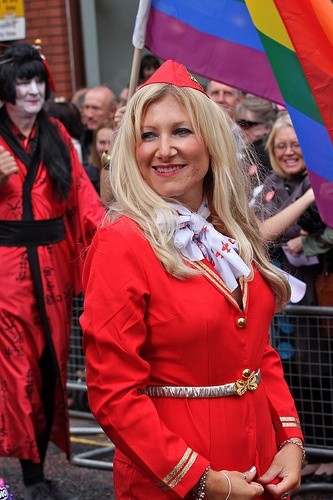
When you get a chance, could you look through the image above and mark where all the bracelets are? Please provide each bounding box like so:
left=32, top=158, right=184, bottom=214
left=242, top=473, right=247, bottom=479
left=198, top=466, right=210, bottom=500
left=219, top=470, right=231, bottom=500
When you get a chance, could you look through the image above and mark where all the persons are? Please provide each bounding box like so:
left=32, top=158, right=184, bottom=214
left=47, top=54, right=333, bottom=484
left=0, top=36, right=106, bottom=492
left=79, top=59, right=307, bottom=500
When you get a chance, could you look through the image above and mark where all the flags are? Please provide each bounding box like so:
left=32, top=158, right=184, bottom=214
left=132, top=0, right=333, bottom=226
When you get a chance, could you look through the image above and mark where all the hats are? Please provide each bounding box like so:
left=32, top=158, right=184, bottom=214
left=136, top=59, right=211, bottom=98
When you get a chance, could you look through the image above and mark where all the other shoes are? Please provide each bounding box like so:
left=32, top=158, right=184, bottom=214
left=21, top=482, right=70, bottom=500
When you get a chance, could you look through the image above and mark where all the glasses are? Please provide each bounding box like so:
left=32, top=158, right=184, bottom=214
left=235, top=119, right=265, bottom=130
